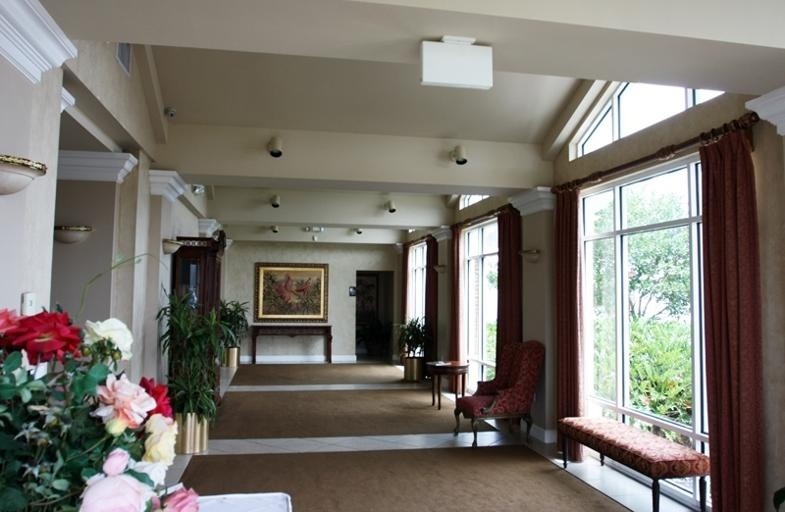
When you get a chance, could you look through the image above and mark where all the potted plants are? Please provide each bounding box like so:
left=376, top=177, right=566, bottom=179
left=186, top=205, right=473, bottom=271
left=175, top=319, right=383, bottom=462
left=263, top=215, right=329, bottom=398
left=214, top=299, right=250, bottom=369
left=158, top=284, right=221, bottom=453
left=398, top=320, right=429, bottom=383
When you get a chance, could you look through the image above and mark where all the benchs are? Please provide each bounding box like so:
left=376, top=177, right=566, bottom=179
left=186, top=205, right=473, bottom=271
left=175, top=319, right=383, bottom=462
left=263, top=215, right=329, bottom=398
left=560, top=412, right=713, bottom=512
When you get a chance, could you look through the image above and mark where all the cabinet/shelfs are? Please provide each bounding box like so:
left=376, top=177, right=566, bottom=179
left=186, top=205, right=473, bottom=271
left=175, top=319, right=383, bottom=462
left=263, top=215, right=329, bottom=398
left=172, top=232, right=223, bottom=409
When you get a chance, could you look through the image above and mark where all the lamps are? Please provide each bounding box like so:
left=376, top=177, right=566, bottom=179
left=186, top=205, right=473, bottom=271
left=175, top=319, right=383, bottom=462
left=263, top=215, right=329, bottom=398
left=268, top=225, right=281, bottom=233
left=0, top=154, right=47, bottom=197
left=449, top=144, right=468, bottom=165
left=382, top=199, right=397, bottom=213
left=351, top=227, right=364, bottom=235
left=269, top=194, right=281, bottom=209
left=433, top=263, right=445, bottom=272
left=518, top=248, right=540, bottom=264
left=53, top=225, right=94, bottom=244
left=163, top=239, right=184, bottom=254
left=266, top=136, right=285, bottom=158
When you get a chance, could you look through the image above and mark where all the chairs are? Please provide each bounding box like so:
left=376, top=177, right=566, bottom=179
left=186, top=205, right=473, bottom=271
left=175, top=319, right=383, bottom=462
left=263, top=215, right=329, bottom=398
left=453, top=341, right=543, bottom=447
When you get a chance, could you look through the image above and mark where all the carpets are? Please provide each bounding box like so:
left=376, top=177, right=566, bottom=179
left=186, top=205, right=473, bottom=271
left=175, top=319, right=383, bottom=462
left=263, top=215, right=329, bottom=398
left=175, top=443, right=635, bottom=512
left=210, top=389, right=501, bottom=439
left=228, top=361, right=411, bottom=387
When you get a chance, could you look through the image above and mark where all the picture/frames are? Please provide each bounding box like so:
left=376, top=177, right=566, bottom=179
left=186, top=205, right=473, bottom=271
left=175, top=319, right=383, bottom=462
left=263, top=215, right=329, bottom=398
left=256, top=262, right=330, bottom=322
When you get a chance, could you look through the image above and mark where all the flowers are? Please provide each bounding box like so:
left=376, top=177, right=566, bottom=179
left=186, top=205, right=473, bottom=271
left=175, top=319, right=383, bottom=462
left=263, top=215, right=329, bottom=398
left=0, top=308, right=197, bottom=511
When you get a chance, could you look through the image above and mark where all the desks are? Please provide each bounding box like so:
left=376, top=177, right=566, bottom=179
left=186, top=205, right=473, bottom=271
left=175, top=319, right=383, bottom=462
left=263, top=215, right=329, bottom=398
left=428, top=359, right=469, bottom=407
left=255, top=325, right=333, bottom=366
